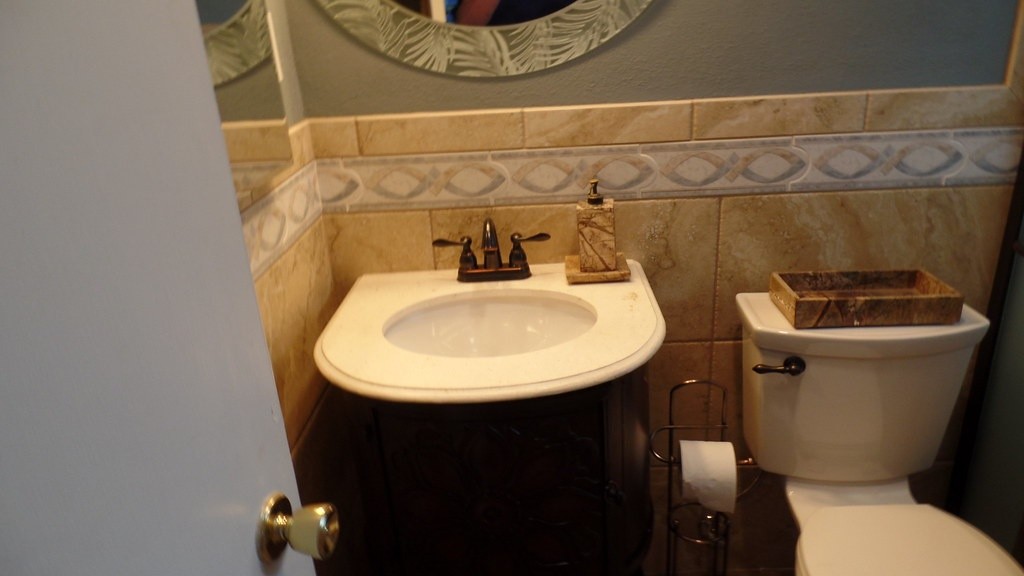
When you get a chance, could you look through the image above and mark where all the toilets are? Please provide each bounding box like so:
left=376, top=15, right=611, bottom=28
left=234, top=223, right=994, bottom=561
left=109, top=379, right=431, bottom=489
left=736, top=287, right=1024, bottom=576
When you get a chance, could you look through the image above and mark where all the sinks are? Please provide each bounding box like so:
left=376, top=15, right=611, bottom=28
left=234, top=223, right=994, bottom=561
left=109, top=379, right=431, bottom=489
left=313, top=282, right=667, bottom=403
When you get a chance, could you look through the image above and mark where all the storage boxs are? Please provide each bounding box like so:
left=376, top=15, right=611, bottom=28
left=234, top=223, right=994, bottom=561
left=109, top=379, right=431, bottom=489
left=769, top=266, right=965, bottom=330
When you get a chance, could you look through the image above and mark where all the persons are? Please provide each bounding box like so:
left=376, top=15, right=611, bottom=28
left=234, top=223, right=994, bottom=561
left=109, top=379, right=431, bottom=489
left=445, top=0, right=576, bottom=27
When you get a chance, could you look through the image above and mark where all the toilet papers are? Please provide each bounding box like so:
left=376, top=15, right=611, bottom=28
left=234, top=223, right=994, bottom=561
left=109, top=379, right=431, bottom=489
left=677, top=438, right=738, bottom=517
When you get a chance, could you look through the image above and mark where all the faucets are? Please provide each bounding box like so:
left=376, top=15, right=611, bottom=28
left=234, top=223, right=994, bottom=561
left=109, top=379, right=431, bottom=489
left=480, top=217, right=503, bottom=269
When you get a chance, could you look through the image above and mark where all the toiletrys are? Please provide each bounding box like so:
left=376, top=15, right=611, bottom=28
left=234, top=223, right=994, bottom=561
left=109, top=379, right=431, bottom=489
left=576, top=179, right=618, bottom=272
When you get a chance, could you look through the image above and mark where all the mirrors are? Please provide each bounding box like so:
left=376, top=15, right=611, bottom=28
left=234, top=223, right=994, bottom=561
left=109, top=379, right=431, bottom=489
left=195, top=0, right=295, bottom=200
left=195, top=0, right=273, bottom=88
left=314, top=0, right=656, bottom=78
